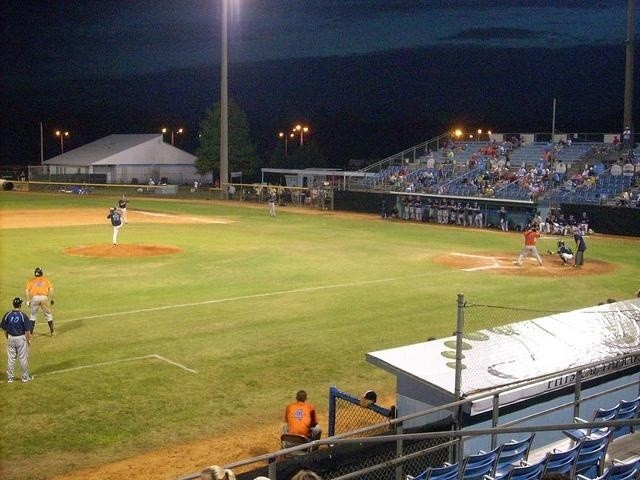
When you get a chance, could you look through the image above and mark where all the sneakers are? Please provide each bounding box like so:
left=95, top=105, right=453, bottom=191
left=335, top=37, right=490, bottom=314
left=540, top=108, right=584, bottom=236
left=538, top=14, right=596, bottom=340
left=7, top=378, right=14, bottom=382
left=22, top=376, right=34, bottom=382
left=51, top=332, right=56, bottom=337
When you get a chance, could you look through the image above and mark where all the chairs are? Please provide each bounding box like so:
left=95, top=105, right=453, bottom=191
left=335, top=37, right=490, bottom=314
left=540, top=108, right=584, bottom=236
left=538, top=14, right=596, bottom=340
left=408, top=395, right=640, bottom=479
left=356, top=141, right=640, bottom=206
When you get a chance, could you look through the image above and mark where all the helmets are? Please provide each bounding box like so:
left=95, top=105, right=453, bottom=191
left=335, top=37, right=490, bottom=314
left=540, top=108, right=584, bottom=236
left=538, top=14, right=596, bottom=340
left=35, top=268, right=43, bottom=276
left=13, top=298, right=24, bottom=305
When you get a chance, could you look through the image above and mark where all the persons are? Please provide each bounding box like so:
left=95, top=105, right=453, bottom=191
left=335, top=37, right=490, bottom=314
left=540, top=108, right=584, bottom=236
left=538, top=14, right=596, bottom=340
left=280, top=390, right=321, bottom=452
left=25, top=267, right=56, bottom=339
left=0, top=298, right=33, bottom=383
left=148, top=176, right=329, bottom=217
left=387, top=125, right=639, bottom=267
left=107, top=195, right=130, bottom=247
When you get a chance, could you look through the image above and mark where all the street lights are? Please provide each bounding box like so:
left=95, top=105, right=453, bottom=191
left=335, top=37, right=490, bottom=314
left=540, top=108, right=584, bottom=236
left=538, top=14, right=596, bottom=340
left=296, top=125, right=308, bottom=146
left=162, top=127, right=183, bottom=145
left=279, top=132, right=295, bottom=156
left=56, top=131, right=68, bottom=154
